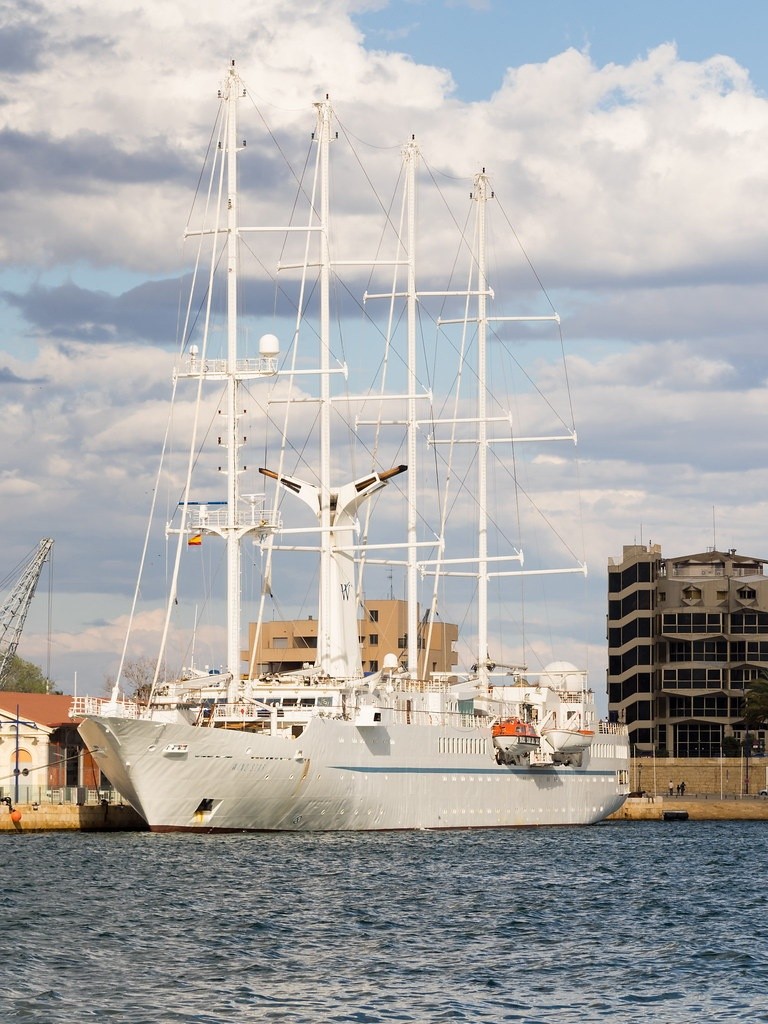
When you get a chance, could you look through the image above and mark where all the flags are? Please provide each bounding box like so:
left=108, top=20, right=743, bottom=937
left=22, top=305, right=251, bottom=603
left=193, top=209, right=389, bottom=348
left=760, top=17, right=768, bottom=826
left=187, top=533, right=202, bottom=546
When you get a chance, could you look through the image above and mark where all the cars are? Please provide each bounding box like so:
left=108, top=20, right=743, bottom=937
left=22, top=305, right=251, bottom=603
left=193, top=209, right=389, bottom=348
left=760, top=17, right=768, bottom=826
left=758, top=789, right=768, bottom=796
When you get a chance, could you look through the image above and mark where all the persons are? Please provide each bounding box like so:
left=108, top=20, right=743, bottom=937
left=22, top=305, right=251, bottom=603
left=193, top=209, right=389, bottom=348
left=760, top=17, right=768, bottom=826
left=604, top=716, right=609, bottom=734
left=677, top=785, right=680, bottom=796
left=680, top=782, right=686, bottom=796
left=669, top=781, right=674, bottom=796
left=599, top=719, right=604, bottom=734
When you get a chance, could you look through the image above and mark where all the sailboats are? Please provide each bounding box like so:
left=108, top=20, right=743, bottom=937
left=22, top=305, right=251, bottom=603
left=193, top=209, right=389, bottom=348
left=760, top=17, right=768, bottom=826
left=66, top=57, right=631, bottom=835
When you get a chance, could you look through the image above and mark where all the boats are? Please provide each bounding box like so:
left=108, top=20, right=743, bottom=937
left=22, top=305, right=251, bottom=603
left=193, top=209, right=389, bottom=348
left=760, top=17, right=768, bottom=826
left=541, top=727, right=595, bottom=752
left=492, top=717, right=540, bottom=757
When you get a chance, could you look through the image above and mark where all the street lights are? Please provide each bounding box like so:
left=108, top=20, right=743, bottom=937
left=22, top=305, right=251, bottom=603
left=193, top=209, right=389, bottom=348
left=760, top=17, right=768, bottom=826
left=0, top=704, right=37, bottom=804
left=637, top=762, right=645, bottom=792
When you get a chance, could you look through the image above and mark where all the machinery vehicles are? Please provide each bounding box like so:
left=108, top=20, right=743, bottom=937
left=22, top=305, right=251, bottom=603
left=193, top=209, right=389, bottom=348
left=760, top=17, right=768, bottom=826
left=0, top=535, right=58, bottom=694
